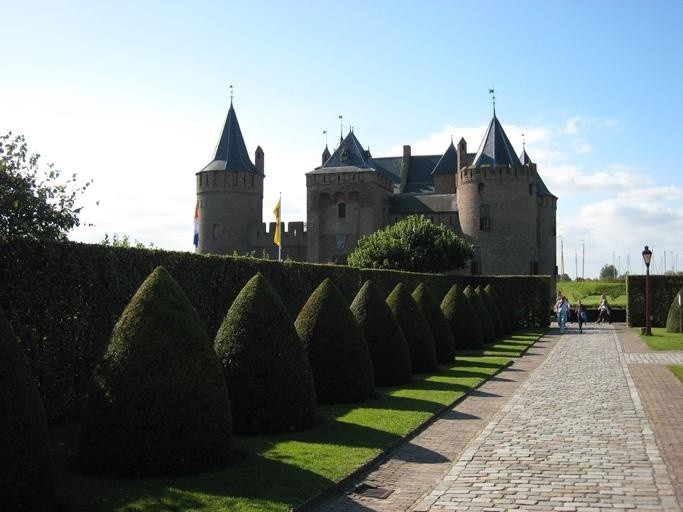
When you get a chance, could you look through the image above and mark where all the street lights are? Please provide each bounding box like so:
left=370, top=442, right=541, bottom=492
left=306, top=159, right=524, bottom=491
left=641, top=245, right=653, bottom=336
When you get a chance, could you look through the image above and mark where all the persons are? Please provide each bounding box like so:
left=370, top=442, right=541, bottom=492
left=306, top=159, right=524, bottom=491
left=555, top=288, right=586, bottom=333
left=596, top=295, right=612, bottom=324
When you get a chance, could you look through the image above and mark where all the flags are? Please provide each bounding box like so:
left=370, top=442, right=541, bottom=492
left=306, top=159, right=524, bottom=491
left=274, top=201, right=281, bottom=251
left=194, top=202, right=199, bottom=247
